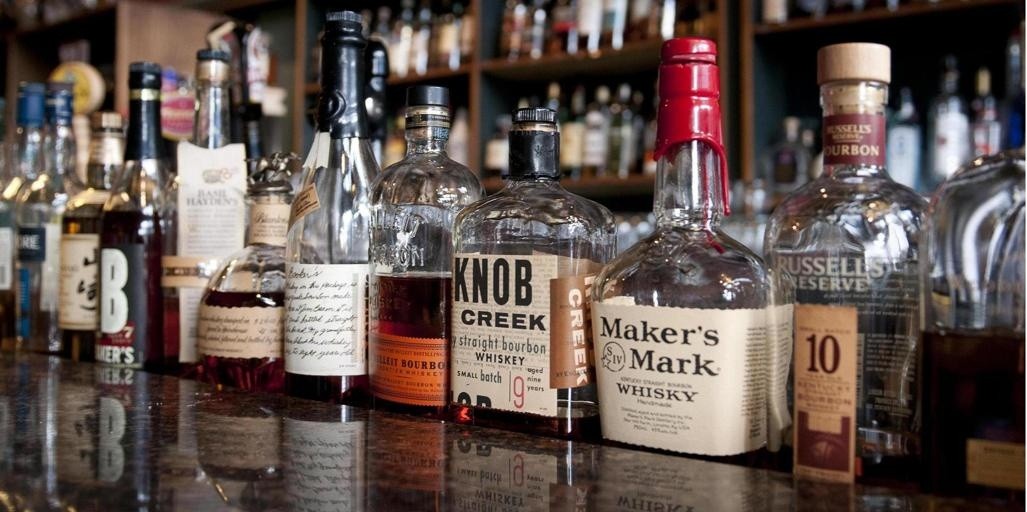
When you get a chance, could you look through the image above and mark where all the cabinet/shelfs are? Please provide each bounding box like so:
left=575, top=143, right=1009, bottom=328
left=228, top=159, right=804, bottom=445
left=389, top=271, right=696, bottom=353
left=227, top=0, right=1025, bottom=220
left=3, top=1, right=223, bottom=143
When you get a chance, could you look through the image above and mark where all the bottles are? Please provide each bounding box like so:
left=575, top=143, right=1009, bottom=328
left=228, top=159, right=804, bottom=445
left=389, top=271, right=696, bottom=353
left=357, top=1, right=475, bottom=72
left=1, top=341, right=929, bottom=510
left=97, top=62, right=181, bottom=371
left=58, top=111, right=151, bottom=364
left=887, top=86, right=920, bottom=191
left=484, top=82, right=670, bottom=182
left=971, top=69, right=999, bottom=158
left=368, top=87, right=489, bottom=420
left=920, top=34, right=1025, bottom=502
left=501, top=0, right=679, bottom=61
left=999, top=36, right=1024, bottom=151
left=928, top=57, right=970, bottom=191
left=762, top=43, right=939, bottom=481
left=450, top=107, right=619, bottom=441
left=760, top=116, right=808, bottom=191
left=284, top=12, right=405, bottom=402
left=15, top=80, right=86, bottom=355
left=145, top=49, right=272, bottom=380
left=198, top=181, right=333, bottom=393
left=590, top=40, right=794, bottom=458
left=0, top=83, right=64, bottom=352
